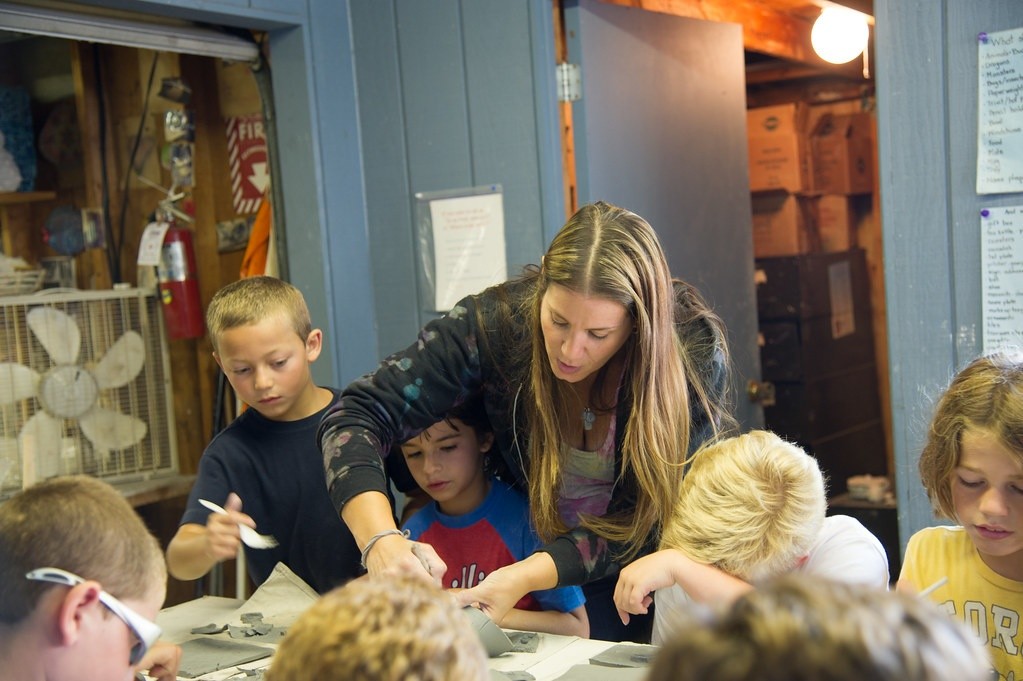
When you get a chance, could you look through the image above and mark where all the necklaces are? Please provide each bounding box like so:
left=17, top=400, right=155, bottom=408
left=564, top=381, right=618, bottom=431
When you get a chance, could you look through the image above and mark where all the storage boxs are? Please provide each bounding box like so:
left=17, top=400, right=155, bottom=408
left=747, top=76, right=901, bottom=584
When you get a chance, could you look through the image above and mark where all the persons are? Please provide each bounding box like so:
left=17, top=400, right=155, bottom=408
left=614, top=430, right=891, bottom=650
left=263, top=571, right=492, bottom=680
left=892, top=349, right=1022, bottom=680
left=0, top=473, right=183, bottom=680
left=398, top=391, right=591, bottom=642
left=316, top=199, right=728, bottom=645
left=640, top=568, right=992, bottom=681
left=162, top=274, right=435, bottom=598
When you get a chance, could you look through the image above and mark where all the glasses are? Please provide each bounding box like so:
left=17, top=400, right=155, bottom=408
left=24, top=566, right=162, bottom=667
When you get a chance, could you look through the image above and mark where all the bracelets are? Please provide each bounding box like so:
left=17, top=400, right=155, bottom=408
left=361, top=528, right=412, bottom=569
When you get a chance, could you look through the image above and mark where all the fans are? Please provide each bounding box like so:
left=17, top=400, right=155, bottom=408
left=0, top=288, right=180, bottom=505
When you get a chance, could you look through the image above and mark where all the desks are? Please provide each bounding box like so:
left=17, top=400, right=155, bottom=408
left=134, top=596, right=662, bottom=680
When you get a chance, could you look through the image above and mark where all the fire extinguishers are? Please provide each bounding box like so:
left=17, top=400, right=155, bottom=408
left=136, top=191, right=207, bottom=343
